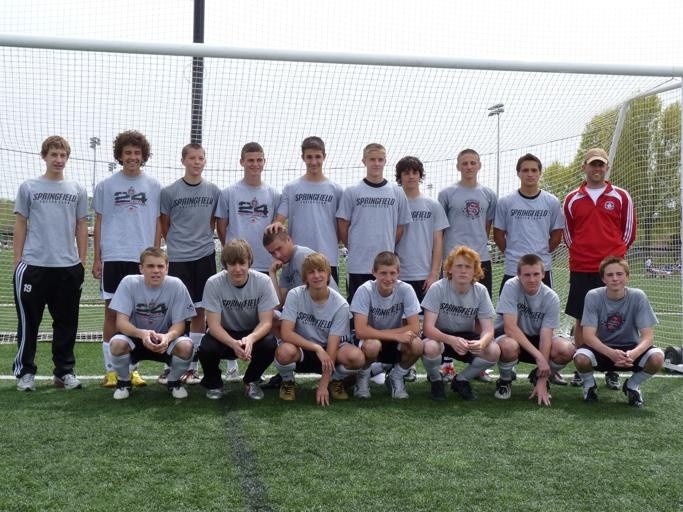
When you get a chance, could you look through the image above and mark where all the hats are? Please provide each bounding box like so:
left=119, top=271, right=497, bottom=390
left=585, top=148, right=609, bottom=164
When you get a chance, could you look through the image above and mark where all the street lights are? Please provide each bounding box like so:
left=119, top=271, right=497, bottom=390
left=89, top=134, right=101, bottom=239
left=107, top=161, right=116, bottom=174
left=425, top=182, right=437, bottom=200
left=486, top=100, right=506, bottom=196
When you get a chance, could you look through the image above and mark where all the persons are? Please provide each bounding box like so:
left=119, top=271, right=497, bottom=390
left=259, top=227, right=340, bottom=389
left=91, top=129, right=162, bottom=388
left=157, top=142, right=221, bottom=385
left=11, top=135, right=90, bottom=391
left=493, top=152, right=568, bottom=385
left=348, top=251, right=424, bottom=399
left=437, top=148, right=497, bottom=383
left=215, top=141, right=283, bottom=381
left=264, top=134, right=344, bottom=284
left=196, top=238, right=281, bottom=400
left=572, top=255, right=665, bottom=405
left=393, top=155, right=450, bottom=381
left=274, top=253, right=366, bottom=407
left=420, top=244, right=501, bottom=401
left=335, top=142, right=411, bottom=328
left=561, top=146, right=637, bottom=390
left=107, top=247, right=198, bottom=400
left=490, top=254, right=575, bottom=407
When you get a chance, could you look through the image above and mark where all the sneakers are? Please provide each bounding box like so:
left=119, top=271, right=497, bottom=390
left=430, top=366, right=516, bottom=401
left=17, top=373, right=81, bottom=392
left=329, top=379, right=371, bottom=400
left=157, top=368, right=296, bottom=401
left=550, top=371, right=644, bottom=407
left=385, top=369, right=416, bottom=400
left=104, top=371, right=147, bottom=400
